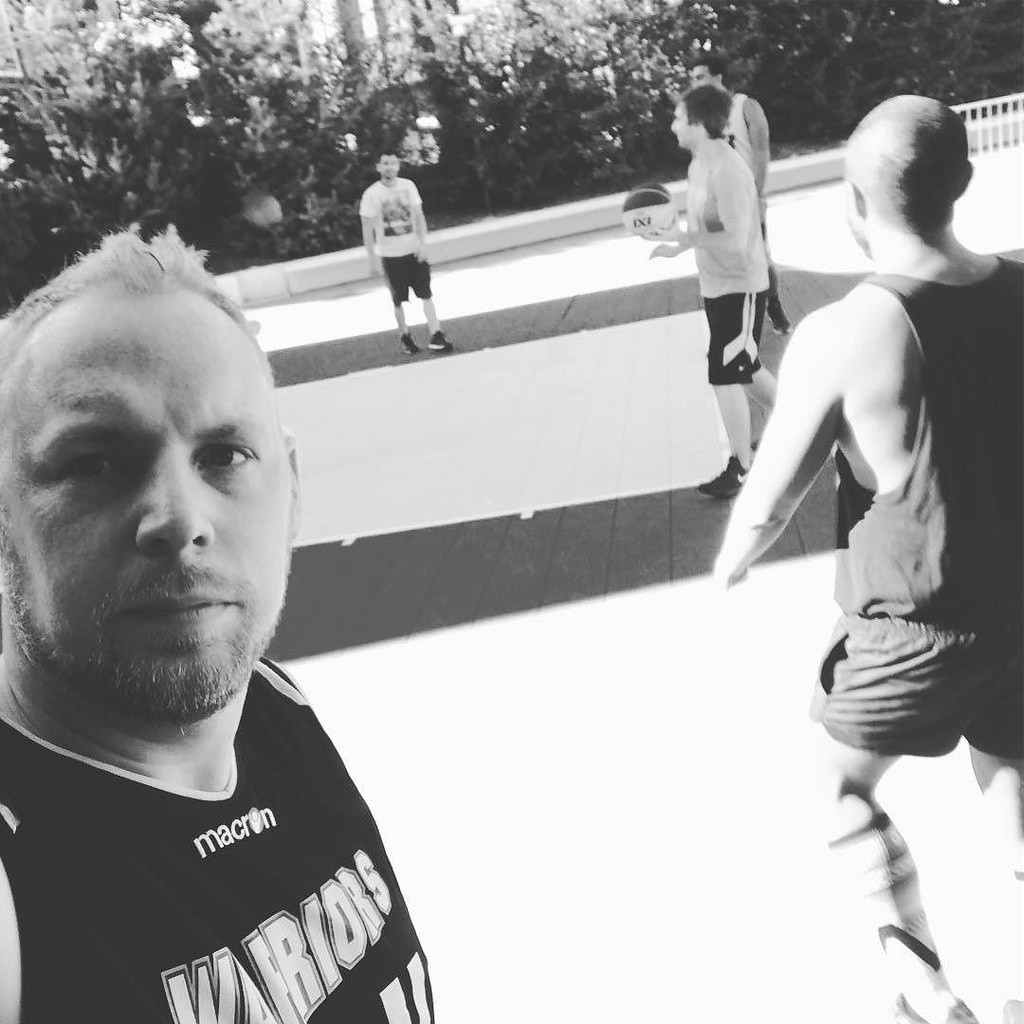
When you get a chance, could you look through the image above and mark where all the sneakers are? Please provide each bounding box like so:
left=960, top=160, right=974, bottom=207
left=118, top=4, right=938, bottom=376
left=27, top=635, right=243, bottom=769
left=766, top=306, right=792, bottom=335
left=400, top=332, right=420, bottom=354
left=428, top=331, right=452, bottom=351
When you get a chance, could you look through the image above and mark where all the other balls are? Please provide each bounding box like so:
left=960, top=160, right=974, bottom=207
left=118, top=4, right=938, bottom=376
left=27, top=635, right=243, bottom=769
left=617, top=180, right=680, bottom=242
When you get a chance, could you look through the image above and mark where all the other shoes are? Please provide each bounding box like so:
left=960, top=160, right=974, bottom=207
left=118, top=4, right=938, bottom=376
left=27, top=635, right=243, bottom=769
left=698, top=456, right=746, bottom=497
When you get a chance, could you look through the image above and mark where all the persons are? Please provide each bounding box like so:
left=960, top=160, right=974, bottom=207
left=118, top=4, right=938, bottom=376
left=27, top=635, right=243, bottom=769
left=714, top=95, right=1024, bottom=1024
left=0, top=230, right=435, bottom=1024
left=359, top=152, right=453, bottom=353
left=685, top=57, right=791, bottom=335
left=649, top=85, right=777, bottom=497
